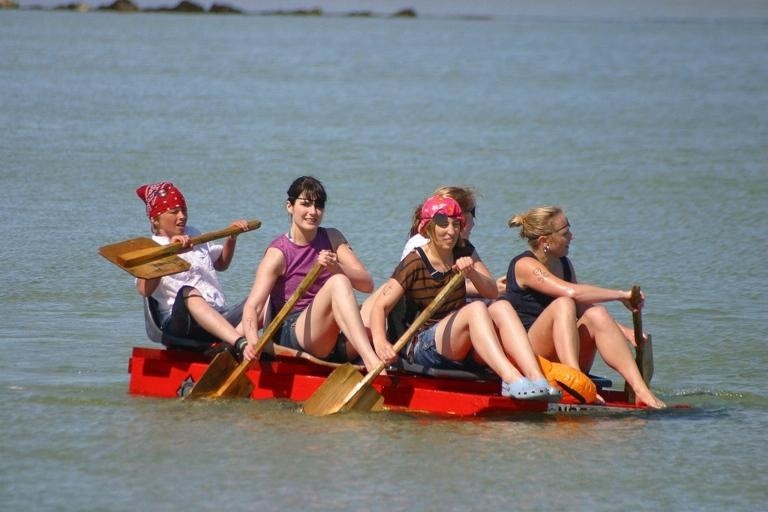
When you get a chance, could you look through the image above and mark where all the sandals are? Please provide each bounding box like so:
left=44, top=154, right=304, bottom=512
left=502, top=379, right=546, bottom=399
left=530, top=377, right=565, bottom=397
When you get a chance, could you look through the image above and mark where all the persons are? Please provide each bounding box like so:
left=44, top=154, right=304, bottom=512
left=135, top=182, right=267, bottom=362
left=401, top=185, right=507, bottom=297
left=372, top=194, right=563, bottom=400
left=502, top=206, right=667, bottom=409
left=241, top=175, right=389, bottom=376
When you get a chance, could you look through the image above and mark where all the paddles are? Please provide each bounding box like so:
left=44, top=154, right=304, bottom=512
left=302, top=270, right=465, bottom=417
left=97, top=219, right=262, bottom=280
left=625, top=285, right=655, bottom=403
left=183, top=261, right=325, bottom=402
